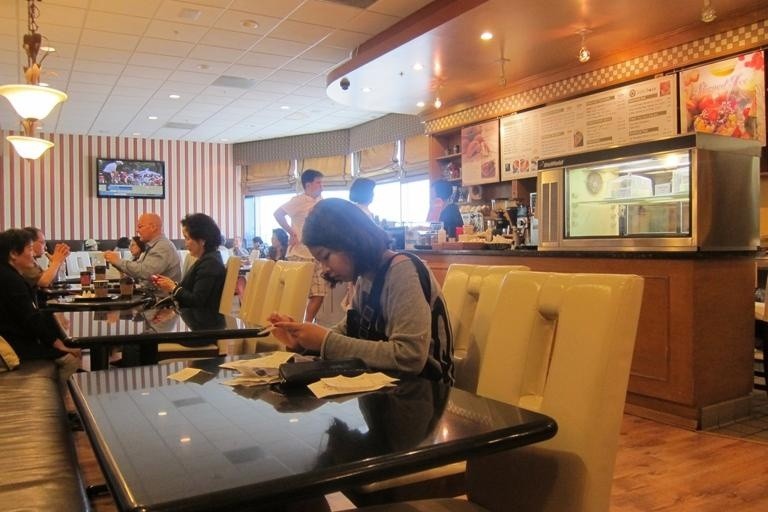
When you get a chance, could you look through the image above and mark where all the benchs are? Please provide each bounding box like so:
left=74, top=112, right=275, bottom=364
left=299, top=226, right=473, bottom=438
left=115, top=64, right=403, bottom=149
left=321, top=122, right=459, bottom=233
left=2, top=351, right=91, bottom=512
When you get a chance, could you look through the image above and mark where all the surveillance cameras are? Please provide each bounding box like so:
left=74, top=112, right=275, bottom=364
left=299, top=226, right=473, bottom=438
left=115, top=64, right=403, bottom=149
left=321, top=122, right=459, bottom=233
left=340, top=78, right=350, bottom=90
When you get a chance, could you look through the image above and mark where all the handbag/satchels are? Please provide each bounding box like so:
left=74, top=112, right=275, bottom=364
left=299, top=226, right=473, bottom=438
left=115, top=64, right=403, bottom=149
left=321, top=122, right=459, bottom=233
left=279, top=359, right=368, bottom=389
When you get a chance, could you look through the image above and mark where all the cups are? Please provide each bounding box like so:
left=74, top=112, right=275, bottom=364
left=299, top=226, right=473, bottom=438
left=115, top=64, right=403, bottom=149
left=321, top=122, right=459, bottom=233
left=78, top=263, right=108, bottom=298
left=452, top=144, right=459, bottom=153
left=118, top=276, right=134, bottom=299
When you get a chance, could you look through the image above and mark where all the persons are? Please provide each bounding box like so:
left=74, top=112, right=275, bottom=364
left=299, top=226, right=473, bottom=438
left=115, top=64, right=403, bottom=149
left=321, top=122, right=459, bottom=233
left=231, top=237, right=248, bottom=257
left=142, top=213, right=225, bottom=365
left=253, top=235, right=268, bottom=257
left=98, top=174, right=162, bottom=185
left=18, top=227, right=72, bottom=287
left=0, top=229, right=81, bottom=359
left=102, top=160, right=123, bottom=192
left=105, top=212, right=183, bottom=300
left=268, top=228, right=288, bottom=261
left=128, top=237, right=143, bottom=260
left=429, top=180, right=465, bottom=242
left=267, top=197, right=456, bottom=384
left=116, top=237, right=130, bottom=249
left=271, top=168, right=329, bottom=322
left=348, top=176, right=377, bottom=223
left=83, top=238, right=102, bottom=251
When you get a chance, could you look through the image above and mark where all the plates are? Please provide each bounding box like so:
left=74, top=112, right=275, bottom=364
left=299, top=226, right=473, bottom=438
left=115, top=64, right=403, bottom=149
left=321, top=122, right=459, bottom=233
left=487, top=242, right=509, bottom=249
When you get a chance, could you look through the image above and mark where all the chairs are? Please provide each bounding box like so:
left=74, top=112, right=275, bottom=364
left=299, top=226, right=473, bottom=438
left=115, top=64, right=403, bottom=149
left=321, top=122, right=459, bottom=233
left=219, top=248, right=329, bottom=346
left=39, top=238, right=190, bottom=284
left=356, top=261, right=651, bottom=509
left=339, top=262, right=533, bottom=512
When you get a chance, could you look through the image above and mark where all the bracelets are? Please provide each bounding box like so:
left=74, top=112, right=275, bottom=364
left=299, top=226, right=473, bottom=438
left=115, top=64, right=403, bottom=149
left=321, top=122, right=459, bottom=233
left=289, top=233, right=297, bottom=241
left=169, top=284, right=176, bottom=294
left=59, top=345, right=66, bottom=352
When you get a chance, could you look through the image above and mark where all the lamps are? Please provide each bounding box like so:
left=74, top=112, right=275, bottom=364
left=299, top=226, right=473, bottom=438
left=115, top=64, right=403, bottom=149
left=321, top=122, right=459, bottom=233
left=574, top=26, right=593, bottom=63
left=2, top=120, right=59, bottom=164
left=492, top=58, right=511, bottom=89
left=700, top=0, right=716, bottom=22
left=2, top=0, right=71, bottom=121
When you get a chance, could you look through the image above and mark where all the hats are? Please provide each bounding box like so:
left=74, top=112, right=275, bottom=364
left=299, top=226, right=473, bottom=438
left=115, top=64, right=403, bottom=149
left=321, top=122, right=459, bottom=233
left=85, top=239, right=100, bottom=247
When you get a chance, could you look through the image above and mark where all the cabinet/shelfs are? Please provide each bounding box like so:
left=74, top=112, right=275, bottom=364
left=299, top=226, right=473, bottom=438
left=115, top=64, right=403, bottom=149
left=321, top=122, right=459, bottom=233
left=535, top=131, right=762, bottom=251
left=431, top=135, right=461, bottom=183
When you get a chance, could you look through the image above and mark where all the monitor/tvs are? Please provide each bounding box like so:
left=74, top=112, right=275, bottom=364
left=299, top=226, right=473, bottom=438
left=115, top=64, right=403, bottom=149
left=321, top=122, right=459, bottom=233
left=386, top=227, right=405, bottom=249
left=96, top=158, right=166, bottom=199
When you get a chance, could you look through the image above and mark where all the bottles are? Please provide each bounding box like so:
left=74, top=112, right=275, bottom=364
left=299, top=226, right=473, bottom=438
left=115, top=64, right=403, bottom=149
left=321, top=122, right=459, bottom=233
left=449, top=185, right=466, bottom=202
